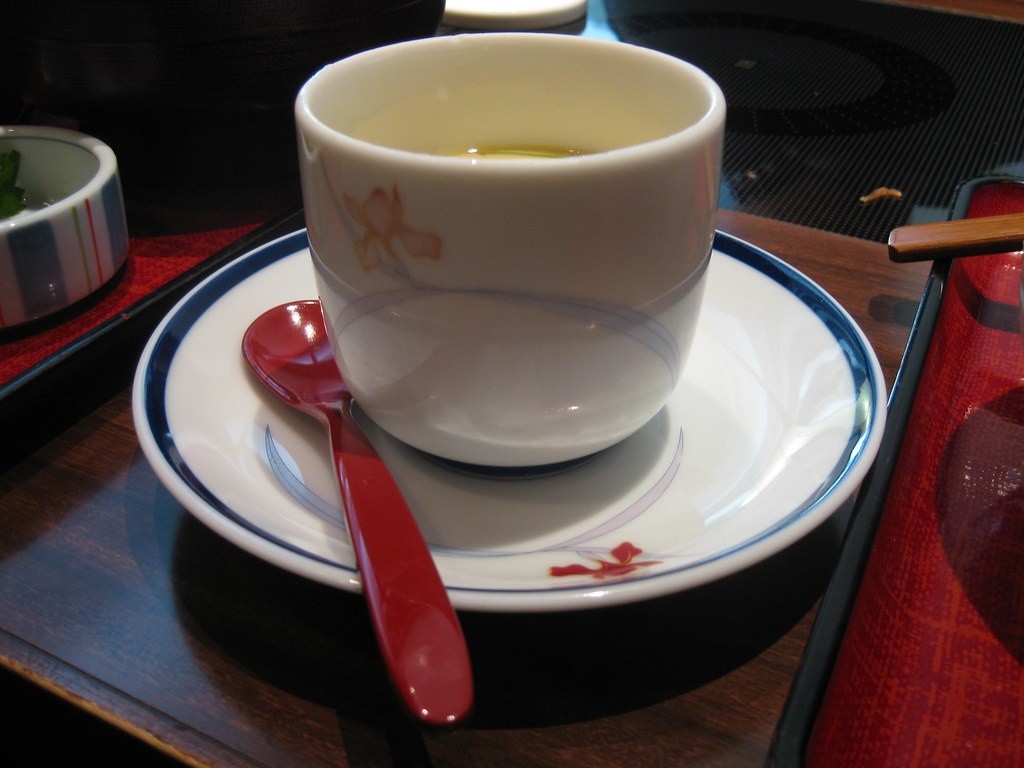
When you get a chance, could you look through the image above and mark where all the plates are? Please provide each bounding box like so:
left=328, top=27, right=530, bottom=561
left=129, top=222, right=890, bottom=613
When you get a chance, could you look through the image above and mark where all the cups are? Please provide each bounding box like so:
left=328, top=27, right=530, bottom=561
left=294, top=28, right=726, bottom=470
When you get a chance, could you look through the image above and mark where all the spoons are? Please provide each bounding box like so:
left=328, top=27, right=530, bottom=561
left=242, top=299, right=476, bottom=725
